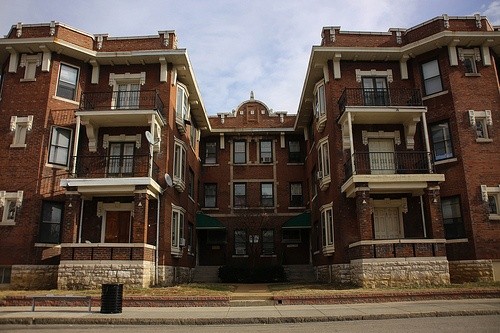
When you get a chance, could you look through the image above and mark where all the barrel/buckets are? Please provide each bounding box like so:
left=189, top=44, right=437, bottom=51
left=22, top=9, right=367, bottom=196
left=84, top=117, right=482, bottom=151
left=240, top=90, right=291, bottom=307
left=101, top=284, right=123, bottom=313
